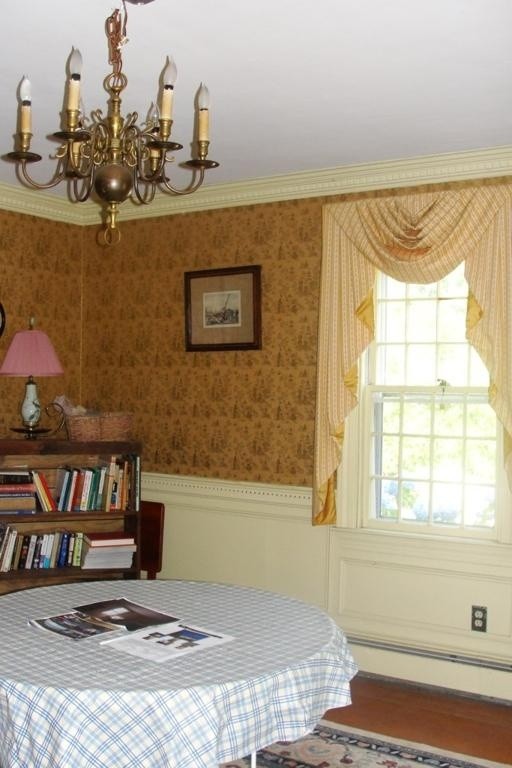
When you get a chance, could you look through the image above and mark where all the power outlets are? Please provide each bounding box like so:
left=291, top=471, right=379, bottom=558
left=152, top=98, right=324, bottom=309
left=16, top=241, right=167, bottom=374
left=470, top=605, right=487, bottom=631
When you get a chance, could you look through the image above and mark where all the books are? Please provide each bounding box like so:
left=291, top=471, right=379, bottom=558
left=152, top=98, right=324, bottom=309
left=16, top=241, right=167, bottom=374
left=0, top=455, right=138, bottom=574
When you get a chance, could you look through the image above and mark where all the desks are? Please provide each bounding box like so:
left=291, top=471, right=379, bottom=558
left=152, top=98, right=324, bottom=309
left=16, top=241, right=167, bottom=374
left=0, top=576, right=365, bottom=768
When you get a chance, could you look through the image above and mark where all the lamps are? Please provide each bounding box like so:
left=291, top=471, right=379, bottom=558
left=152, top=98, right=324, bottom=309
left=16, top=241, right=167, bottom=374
left=0, top=313, right=66, bottom=439
left=0, top=0, right=218, bottom=252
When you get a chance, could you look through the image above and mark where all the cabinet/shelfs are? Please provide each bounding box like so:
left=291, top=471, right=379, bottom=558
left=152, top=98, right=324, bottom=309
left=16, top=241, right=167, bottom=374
left=0, top=438, right=145, bottom=594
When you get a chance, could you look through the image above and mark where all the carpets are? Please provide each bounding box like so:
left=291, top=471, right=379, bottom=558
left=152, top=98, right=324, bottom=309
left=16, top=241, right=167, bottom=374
left=221, top=715, right=512, bottom=767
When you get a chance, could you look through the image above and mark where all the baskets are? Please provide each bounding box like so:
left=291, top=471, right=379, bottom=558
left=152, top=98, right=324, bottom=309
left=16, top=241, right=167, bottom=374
left=65, top=410, right=135, bottom=443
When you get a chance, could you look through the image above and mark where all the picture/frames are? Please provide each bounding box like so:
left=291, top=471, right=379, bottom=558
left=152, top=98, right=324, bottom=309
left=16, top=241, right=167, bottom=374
left=184, top=263, right=263, bottom=349
